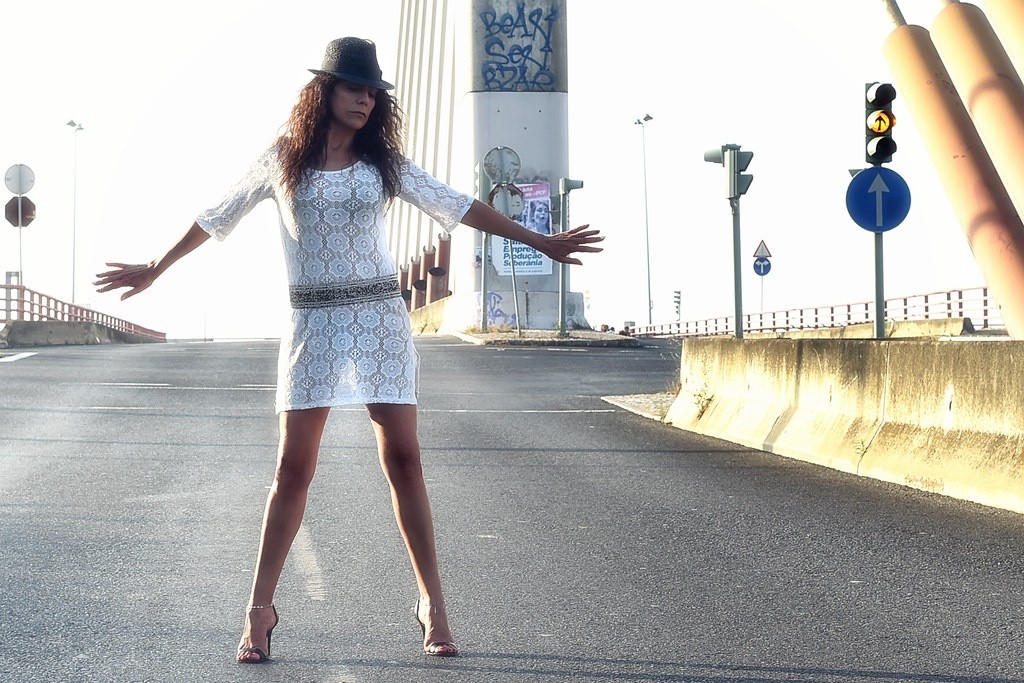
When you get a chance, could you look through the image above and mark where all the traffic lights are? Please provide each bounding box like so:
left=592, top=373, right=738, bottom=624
left=724, top=148, right=754, bottom=198
left=862, top=81, right=899, bottom=165
left=558, top=177, right=584, bottom=196
left=703, top=144, right=725, bottom=168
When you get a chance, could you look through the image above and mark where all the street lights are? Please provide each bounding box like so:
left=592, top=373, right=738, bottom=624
left=66, top=119, right=85, bottom=305
left=634, top=113, right=654, bottom=325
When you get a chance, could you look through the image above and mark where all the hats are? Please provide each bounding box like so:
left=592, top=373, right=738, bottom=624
left=306, top=36, right=396, bottom=90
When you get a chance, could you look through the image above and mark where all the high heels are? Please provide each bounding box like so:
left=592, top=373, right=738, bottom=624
left=413, top=599, right=458, bottom=656
left=235, top=603, right=279, bottom=663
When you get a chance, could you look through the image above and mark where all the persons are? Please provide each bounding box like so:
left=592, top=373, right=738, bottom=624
left=94, top=35, right=607, bottom=664
